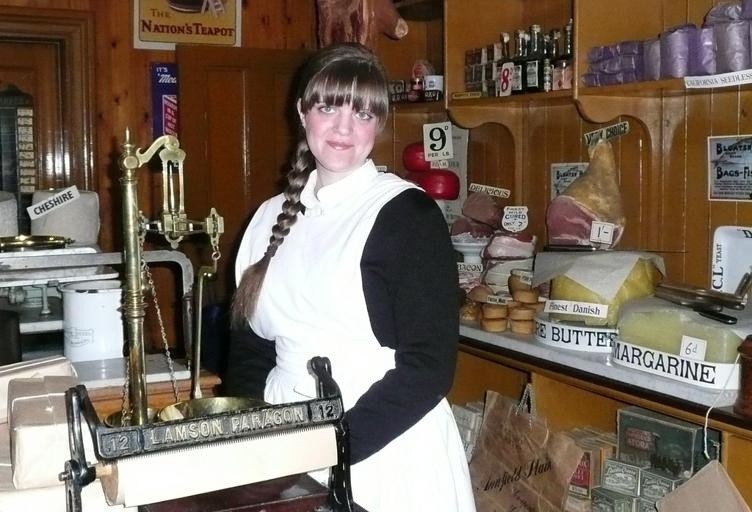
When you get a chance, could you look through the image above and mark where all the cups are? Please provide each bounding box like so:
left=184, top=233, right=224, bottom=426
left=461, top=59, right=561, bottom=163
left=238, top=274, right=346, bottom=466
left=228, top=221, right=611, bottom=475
left=422, top=75, right=443, bottom=95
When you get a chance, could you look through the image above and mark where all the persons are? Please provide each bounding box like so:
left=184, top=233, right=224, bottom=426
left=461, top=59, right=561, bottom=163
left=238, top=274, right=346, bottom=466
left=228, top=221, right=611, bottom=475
left=220, top=43, right=477, bottom=512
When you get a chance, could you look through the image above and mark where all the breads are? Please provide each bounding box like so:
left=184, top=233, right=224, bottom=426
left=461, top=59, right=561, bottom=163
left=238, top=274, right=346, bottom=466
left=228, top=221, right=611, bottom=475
left=459, top=269, right=546, bottom=335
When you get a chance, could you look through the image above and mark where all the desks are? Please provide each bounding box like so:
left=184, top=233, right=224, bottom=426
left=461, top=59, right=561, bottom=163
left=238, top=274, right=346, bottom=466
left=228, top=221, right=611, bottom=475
left=443, top=309, right=750, bottom=510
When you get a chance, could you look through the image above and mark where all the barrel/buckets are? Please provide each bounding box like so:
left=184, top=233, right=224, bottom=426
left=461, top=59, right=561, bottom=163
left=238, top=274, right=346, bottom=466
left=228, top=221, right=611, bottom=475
left=58, top=279, right=123, bottom=362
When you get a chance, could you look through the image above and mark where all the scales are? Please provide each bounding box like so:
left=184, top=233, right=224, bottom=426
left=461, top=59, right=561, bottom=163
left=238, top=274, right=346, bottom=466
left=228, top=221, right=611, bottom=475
left=101, top=126, right=268, bottom=428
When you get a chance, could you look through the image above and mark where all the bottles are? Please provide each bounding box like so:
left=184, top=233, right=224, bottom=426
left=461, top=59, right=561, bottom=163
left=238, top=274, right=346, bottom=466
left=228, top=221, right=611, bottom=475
left=500, top=23, right=573, bottom=95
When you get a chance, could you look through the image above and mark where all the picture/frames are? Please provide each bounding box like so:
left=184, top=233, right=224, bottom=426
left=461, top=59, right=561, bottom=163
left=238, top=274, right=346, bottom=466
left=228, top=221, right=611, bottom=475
left=128, top=0, right=243, bottom=52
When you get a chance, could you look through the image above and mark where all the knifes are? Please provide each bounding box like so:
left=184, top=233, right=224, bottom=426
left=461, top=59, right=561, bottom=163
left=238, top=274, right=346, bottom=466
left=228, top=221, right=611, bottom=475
left=652, top=282, right=745, bottom=325
